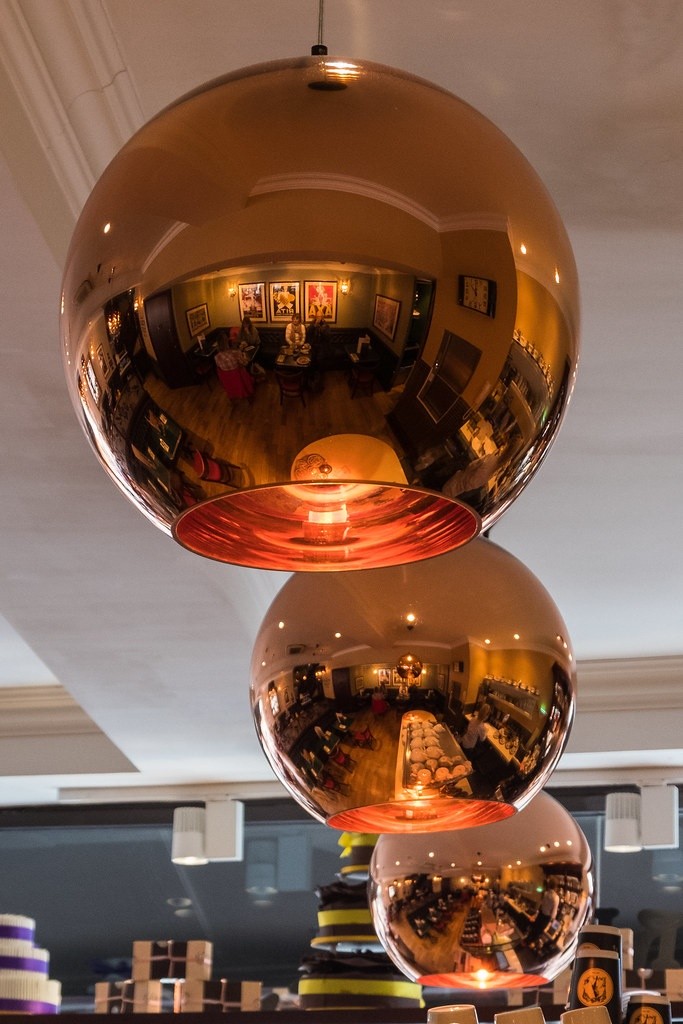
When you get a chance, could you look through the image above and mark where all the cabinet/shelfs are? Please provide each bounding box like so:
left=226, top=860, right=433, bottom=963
left=472, top=675, right=542, bottom=744
left=511, top=661, right=573, bottom=795
left=456, top=332, right=555, bottom=498
left=503, top=863, right=583, bottom=957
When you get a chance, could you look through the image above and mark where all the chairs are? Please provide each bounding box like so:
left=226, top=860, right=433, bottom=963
left=191, top=448, right=242, bottom=490
left=176, top=481, right=202, bottom=512
left=350, top=364, right=378, bottom=400
left=276, top=371, right=307, bottom=408
left=321, top=722, right=377, bottom=797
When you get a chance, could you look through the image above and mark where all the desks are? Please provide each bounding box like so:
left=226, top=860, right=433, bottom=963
left=344, top=343, right=379, bottom=384
left=230, top=340, right=260, bottom=362
left=153, top=411, right=205, bottom=469
left=458, top=712, right=519, bottom=783
left=404, top=891, right=449, bottom=940
left=278, top=698, right=353, bottom=792
left=274, top=345, right=313, bottom=390
left=394, top=710, right=473, bottom=804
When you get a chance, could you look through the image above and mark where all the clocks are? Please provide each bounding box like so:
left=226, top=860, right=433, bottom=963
left=458, top=275, right=497, bottom=318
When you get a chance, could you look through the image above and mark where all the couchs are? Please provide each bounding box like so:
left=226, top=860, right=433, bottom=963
left=123, top=390, right=186, bottom=485
left=186, top=326, right=401, bottom=395
left=355, top=689, right=447, bottom=713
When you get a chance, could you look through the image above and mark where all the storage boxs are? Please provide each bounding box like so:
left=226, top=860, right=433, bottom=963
left=94, top=940, right=262, bottom=1013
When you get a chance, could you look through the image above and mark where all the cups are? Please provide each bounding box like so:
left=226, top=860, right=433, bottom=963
left=560, top=1006, right=613, bottom=1024
left=621, top=993, right=673, bottom=1024
left=427, top=1003, right=479, bottom=1024
left=493, top=1006, right=546, bottom=1024
left=564, top=924, right=623, bottom=1011
left=569, top=948, right=622, bottom=1024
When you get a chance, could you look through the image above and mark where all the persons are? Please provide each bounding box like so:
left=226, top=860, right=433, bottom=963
left=285, top=311, right=306, bottom=347
left=457, top=702, right=493, bottom=772
left=213, top=330, right=258, bottom=391
left=372, top=681, right=388, bottom=702
left=397, top=681, right=408, bottom=696
left=237, top=315, right=260, bottom=349
left=525, top=876, right=560, bottom=947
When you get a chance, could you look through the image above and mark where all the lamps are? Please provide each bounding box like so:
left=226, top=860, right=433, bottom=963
left=306, top=56, right=349, bottom=93
left=366, top=788, right=594, bottom=988
left=396, top=647, right=422, bottom=678
left=249, top=535, right=576, bottom=835
left=170, top=800, right=244, bottom=866
left=604, top=782, right=679, bottom=854
left=407, top=615, right=416, bottom=631
left=60, top=0, right=582, bottom=570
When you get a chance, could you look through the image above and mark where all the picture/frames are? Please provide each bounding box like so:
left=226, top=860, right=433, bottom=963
left=238, top=280, right=338, bottom=324
left=373, top=294, right=401, bottom=343
left=436, top=674, right=445, bottom=691
left=377, top=667, right=421, bottom=686
left=354, top=676, right=365, bottom=690
left=184, top=303, right=211, bottom=341
